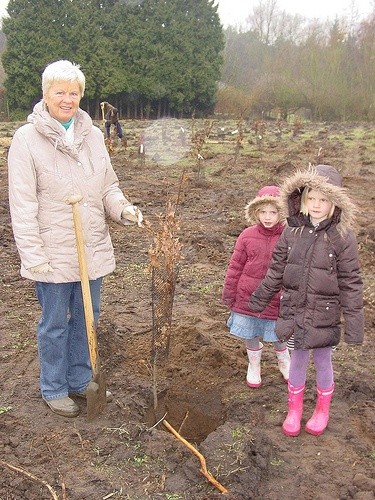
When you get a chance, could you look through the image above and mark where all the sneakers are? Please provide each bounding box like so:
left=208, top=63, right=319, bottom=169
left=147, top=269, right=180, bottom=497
left=72, top=382, right=113, bottom=403
left=45, top=398, right=79, bottom=417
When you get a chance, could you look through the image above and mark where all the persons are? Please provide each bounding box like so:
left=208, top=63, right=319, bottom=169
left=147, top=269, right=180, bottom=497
left=8, top=60, right=144, bottom=417
left=248, top=165, right=364, bottom=437
left=222, top=186, right=292, bottom=388
left=101, top=102, right=125, bottom=140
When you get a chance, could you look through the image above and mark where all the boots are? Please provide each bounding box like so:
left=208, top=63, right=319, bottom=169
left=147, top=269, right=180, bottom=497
left=273, top=347, right=291, bottom=384
left=245, top=341, right=264, bottom=389
left=304, top=382, right=334, bottom=436
left=282, top=380, right=305, bottom=436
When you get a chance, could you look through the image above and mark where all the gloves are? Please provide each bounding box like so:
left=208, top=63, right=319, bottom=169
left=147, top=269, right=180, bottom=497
left=31, top=263, right=54, bottom=273
left=122, top=206, right=143, bottom=223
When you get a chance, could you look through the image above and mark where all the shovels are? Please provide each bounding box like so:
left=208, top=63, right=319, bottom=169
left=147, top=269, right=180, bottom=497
left=65, top=195, right=106, bottom=420
